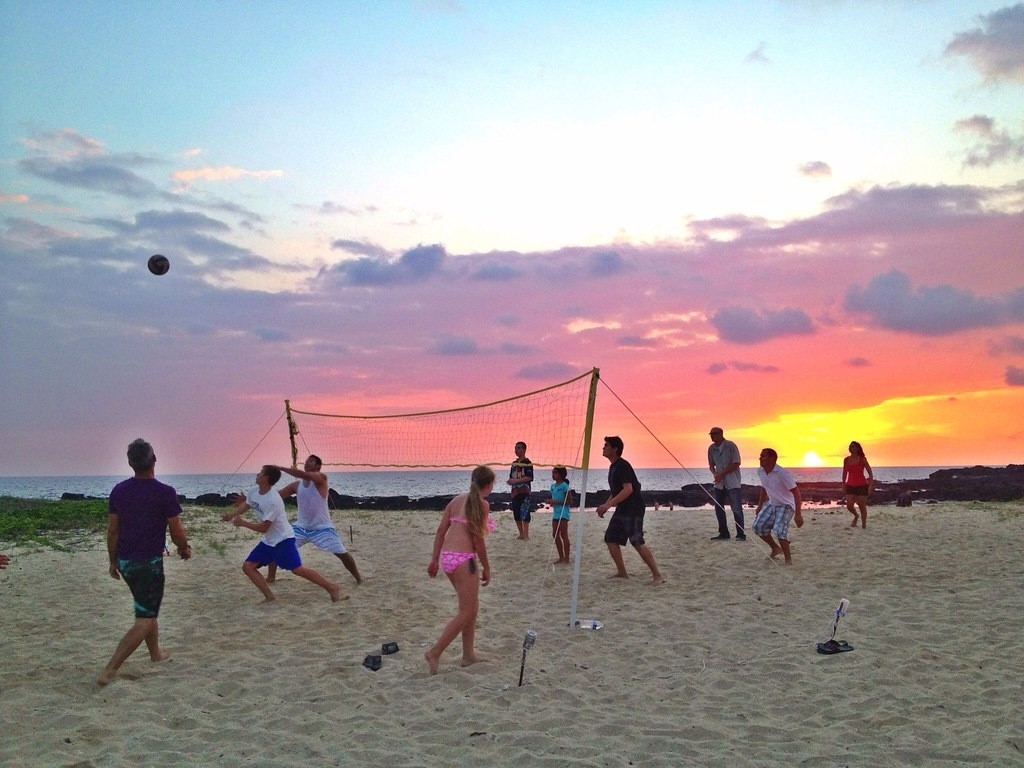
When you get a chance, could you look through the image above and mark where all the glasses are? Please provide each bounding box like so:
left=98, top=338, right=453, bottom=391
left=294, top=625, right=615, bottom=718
left=709, top=433, right=720, bottom=436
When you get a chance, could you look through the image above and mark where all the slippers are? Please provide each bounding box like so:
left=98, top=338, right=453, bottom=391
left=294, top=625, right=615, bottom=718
left=382, top=642, right=399, bottom=655
left=362, top=655, right=382, bottom=670
left=818, top=640, right=853, bottom=654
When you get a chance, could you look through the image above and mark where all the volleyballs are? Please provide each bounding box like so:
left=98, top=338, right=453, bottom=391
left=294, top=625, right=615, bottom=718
left=147, top=253, right=170, bottom=276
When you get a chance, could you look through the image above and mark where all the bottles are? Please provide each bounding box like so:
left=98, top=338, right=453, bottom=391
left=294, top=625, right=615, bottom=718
left=581, top=620, right=605, bottom=630
left=834, top=597, right=850, bottom=626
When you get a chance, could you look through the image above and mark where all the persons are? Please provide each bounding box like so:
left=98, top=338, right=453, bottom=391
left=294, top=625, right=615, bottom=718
left=230, top=455, right=363, bottom=586
left=842, top=441, right=873, bottom=529
left=708, top=427, right=746, bottom=541
left=425, top=466, right=495, bottom=675
left=752, top=448, right=804, bottom=565
left=596, top=436, right=664, bottom=586
left=897, top=489, right=912, bottom=507
left=506, top=442, right=534, bottom=539
left=669, top=500, right=673, bottom=510
left=655, top=500, right=658, bottom=510
left=223, top=465, right=339, bottom=606
left=96, top=438, right=191, bottom=685
left=549, top=464, right=572, bottom=564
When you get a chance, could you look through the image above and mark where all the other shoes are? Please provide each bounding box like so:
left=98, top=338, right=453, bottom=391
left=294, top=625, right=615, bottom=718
left=711, top=534, right=730, bottom=539
left=736, top=535, right=746, bottom=541
